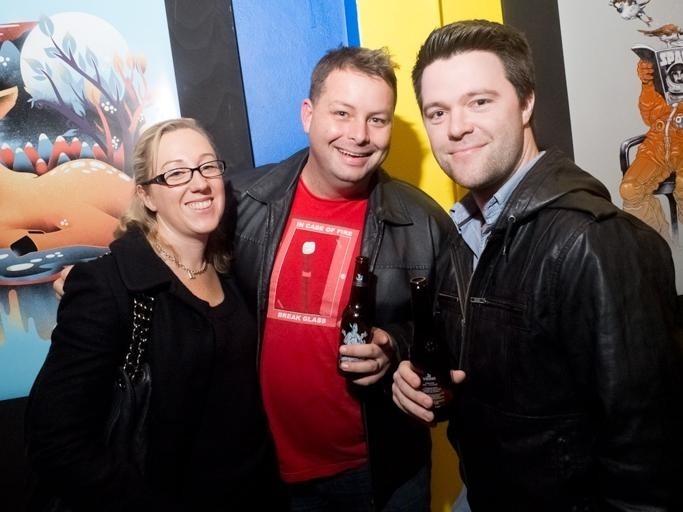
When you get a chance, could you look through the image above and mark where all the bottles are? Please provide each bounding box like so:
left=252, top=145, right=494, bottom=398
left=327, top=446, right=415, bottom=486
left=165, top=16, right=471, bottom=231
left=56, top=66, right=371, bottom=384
left=407, top=275, right=457, bottom=426
left=334, top=254, right=374, bottom=381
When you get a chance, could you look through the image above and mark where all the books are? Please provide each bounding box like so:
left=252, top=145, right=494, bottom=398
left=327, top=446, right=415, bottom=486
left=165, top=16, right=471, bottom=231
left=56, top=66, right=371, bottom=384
left=630, top=44, right=683, bottom=106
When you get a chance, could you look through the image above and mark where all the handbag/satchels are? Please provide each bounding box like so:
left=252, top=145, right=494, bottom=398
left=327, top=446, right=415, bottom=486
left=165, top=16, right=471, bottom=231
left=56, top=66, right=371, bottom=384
left=88, top=351, right=152, bottom=456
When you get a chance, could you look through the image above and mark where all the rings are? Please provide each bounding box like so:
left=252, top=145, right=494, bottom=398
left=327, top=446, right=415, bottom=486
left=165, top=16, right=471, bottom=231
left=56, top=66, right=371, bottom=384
left=373, top=358, right=379, bottom=372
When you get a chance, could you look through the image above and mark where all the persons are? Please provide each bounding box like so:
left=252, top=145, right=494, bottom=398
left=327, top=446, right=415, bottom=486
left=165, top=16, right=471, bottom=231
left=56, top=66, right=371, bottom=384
left=387, top=18, right=683, bottom=512
left=22, top=117, right=264, bottom=511
left=51, top=42, right=457, bottom=511
left=620, top=59, right=683, bottom=249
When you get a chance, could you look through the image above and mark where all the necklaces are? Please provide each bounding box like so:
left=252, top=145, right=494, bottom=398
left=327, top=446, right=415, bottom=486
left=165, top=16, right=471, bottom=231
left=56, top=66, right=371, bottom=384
left=153, top=242, right=207, bottom=279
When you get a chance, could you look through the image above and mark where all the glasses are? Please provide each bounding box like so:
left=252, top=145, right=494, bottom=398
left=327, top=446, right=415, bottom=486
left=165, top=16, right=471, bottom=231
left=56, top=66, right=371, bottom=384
left=138, top=161, right=225, bottom=186
